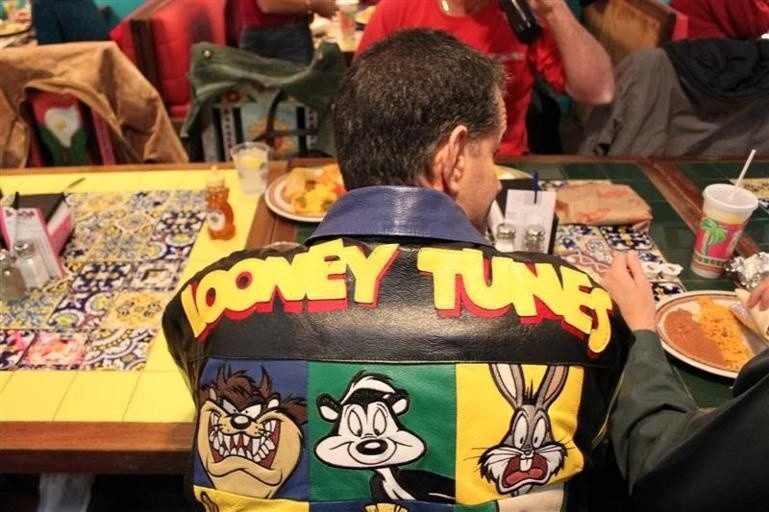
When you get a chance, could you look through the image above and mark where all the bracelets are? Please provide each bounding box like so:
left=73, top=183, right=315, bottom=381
left=306, top=1, right=314, bottom=17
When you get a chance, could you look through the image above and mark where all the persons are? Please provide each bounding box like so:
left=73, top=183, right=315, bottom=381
left=600, top=247, right=767, bottom=511
left=236, top=0, right=343, bottom=70
left=156, top=29, right=629, bottom=509
left=351, top=1, right=614, bottom=159
left=667, top=0, right=769, bottom=43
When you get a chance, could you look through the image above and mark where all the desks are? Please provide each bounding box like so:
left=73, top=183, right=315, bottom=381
left=0, top=155, right=765, bottom=479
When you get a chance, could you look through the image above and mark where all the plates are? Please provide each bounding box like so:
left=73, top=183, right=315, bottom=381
left=264, top=163, right=346, bottom=223
left=651, top=288, right=769, bottom=379
left=354, top=10, right=370, bottom=24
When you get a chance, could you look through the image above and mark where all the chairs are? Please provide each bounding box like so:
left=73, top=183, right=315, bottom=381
left=1, top=0, right=360, bottom=169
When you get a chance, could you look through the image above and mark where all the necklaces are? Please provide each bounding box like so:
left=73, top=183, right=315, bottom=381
left=441, top=0, right=485, bottom=15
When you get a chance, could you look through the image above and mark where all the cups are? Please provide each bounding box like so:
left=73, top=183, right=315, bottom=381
left=335, top=1, right=358, bottom=37
left=228, top=140, right=270, bottom=196
left=690, top=180, right=761, bottom=283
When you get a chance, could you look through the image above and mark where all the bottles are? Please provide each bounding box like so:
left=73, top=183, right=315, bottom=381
left=205, top=164, right=235, bottom=241
left=493, top=222, right=546, bottom=254
left=0, top=236, right=51, bottom=302
